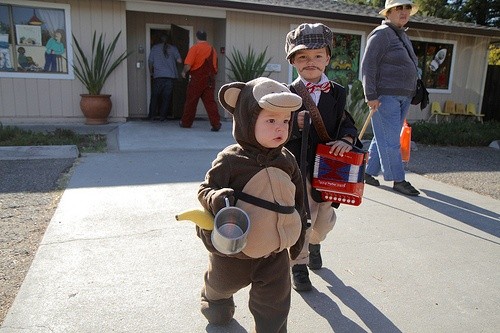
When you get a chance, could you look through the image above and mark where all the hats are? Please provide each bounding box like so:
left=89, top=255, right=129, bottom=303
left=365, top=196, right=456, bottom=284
left=378, top=0, right=419, bottom=16
left=285, top=22, right=334, bottom=65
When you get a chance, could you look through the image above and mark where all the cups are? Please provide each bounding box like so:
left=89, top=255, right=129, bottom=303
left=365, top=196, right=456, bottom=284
left=210, top=198, right=250, bottom=255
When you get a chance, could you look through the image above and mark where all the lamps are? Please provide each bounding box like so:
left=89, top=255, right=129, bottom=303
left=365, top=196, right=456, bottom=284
left=28, top=8, right=43, bottom=25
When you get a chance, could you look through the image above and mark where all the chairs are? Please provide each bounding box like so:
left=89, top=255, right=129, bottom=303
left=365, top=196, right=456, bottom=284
left=427, top=101, right=486, bottom=125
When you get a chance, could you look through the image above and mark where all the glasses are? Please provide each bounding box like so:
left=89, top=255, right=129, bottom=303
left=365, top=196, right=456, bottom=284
left=392, top=4, right=412, bottom=11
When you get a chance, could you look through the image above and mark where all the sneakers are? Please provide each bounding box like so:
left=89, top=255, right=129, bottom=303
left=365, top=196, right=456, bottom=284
left=292, top=263, right=312, bottom=292
left=309, top=243, right=322, bottom=270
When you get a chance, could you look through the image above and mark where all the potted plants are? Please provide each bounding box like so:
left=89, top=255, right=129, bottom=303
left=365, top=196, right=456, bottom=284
left=58, top=30, right=135, bottom=124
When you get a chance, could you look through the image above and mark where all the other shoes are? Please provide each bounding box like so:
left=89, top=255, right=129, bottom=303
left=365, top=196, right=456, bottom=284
left=393, top=181, right=419, bottom=195
left=179, top=120, right=185, bottom=127
left=366, top=175, right=380, bottom=186
left=211, top=124, right=221, bottom=131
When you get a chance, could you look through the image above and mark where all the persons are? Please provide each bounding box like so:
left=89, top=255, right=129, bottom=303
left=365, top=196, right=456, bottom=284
left=149, top=32, right=182, bottom=121
left=194, top=77, right=309, bottom=333
left=360, top=0, right=424, bottom=196
left=180, top=29, right=222, bottom=131
left=284, top=23, right=362, bottom=291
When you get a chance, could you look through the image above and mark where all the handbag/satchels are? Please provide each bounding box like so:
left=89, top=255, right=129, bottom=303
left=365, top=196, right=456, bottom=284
left=400, top=118, right=411, bottom=160
